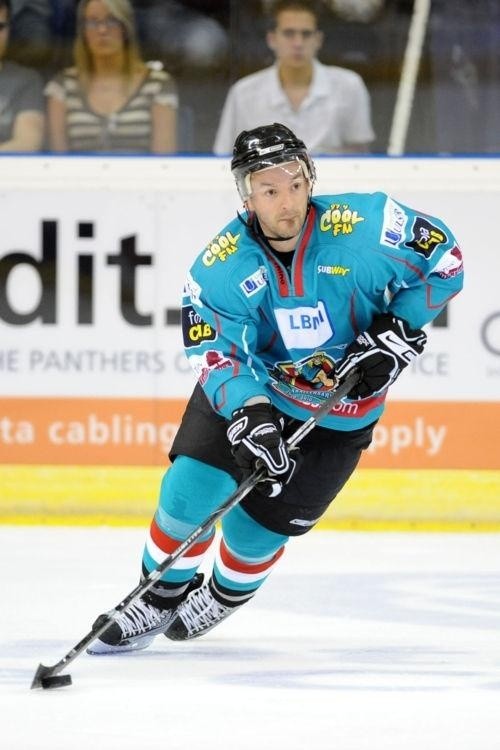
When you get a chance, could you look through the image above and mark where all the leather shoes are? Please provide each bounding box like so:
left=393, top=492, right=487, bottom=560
left=332, top=310, right=426, bottom=402
left=223, top=400, right=304, bottom=498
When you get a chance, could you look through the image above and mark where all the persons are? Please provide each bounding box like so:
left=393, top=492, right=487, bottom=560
left=212, top=3, right=376, bottom=155
left=43, top=0, right=180, bottom=153
left=3, top=6, right=48, bottom=153
left=91, top=124, right=462, bottom=647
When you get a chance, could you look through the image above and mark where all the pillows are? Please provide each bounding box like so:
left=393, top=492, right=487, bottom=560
left=230, top=121, right=317, bottom=199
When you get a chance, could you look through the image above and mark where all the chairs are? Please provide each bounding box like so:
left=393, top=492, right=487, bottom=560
left=164, top=579, right=245, bottom=642
left=90, top=572, right=203, bottom=647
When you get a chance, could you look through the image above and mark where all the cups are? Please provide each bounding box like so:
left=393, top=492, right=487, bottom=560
left=81, top=18, right=122, bottom=29
